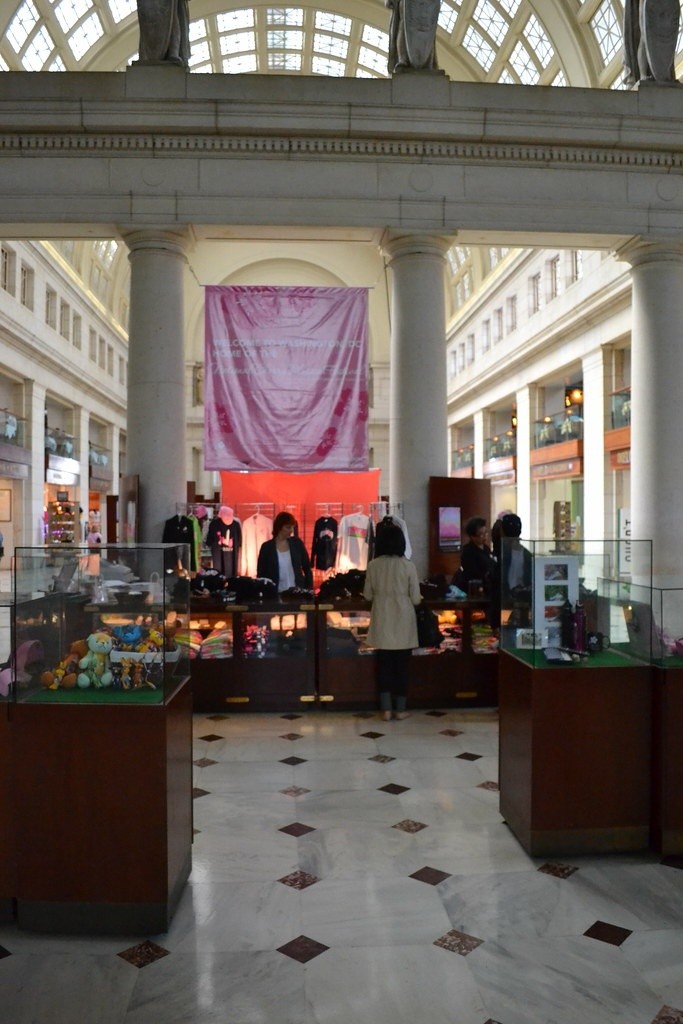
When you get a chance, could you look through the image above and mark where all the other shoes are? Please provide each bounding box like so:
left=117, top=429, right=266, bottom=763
left=396, top=711, right=410, bottom=719
left=383, top=712, right=394, bottom=720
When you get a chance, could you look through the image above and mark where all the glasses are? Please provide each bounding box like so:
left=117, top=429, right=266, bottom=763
left=474, top=533, right=488, bottom=538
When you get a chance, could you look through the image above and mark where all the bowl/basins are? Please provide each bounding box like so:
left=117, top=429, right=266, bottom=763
left=113, top=590, right=150, bottom=606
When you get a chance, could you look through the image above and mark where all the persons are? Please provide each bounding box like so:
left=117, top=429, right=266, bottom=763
left=86, top=523, right=102, bottom=571
left=461, top=517, right=497, bottom=579
left=363, top=526, right=421, bottom=720
left=257, top=512, right=313, bottom=592
left=0, top=407, right=18, bottom=440
left=48, top=427, right=66, bottom=456
left=487, top=514, right=532, bottom=640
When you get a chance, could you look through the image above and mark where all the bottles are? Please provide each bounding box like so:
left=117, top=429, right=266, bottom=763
left=574, top=599, right=587, bottom=652
left=561, top=600, right=575, bottom=650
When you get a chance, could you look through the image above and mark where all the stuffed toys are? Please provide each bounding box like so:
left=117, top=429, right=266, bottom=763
left=40, top=621, right=168, bottom=690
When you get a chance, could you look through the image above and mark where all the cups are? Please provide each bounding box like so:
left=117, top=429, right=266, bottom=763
left=584, top=631, right=610, bottom=653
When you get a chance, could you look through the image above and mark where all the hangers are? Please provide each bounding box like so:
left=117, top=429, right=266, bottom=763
left=187, top=506, right=195, bottom=518
left=321, top=505, right=331, bottom=517
left=253, top=506, right=260, bottom=517
left=356, top=504, right=369, bottom=517
left=176, top=506, right=183, bottom=516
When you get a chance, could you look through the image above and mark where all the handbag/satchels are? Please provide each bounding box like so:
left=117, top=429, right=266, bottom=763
left=97, top=536, right=101, bottom=543
left=450, top=564, right=482, bottom=588
left=415, top=597, right=445, bottom=650
